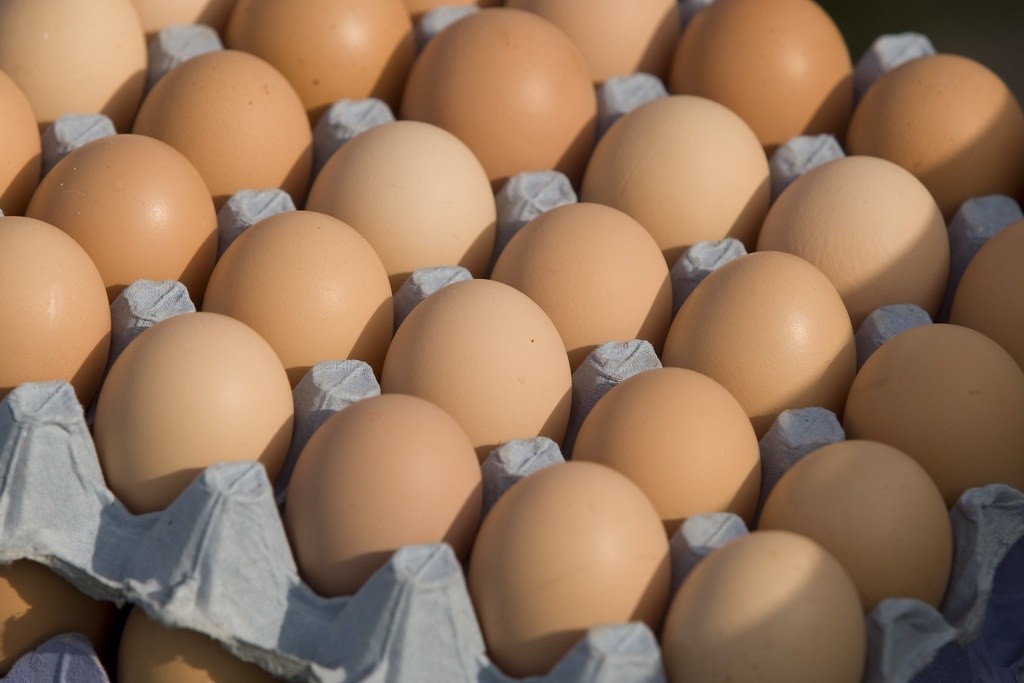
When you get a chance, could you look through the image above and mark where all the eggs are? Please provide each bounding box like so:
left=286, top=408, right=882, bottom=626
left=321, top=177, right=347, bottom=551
left=1, top=1, right=1024, bottom=683
